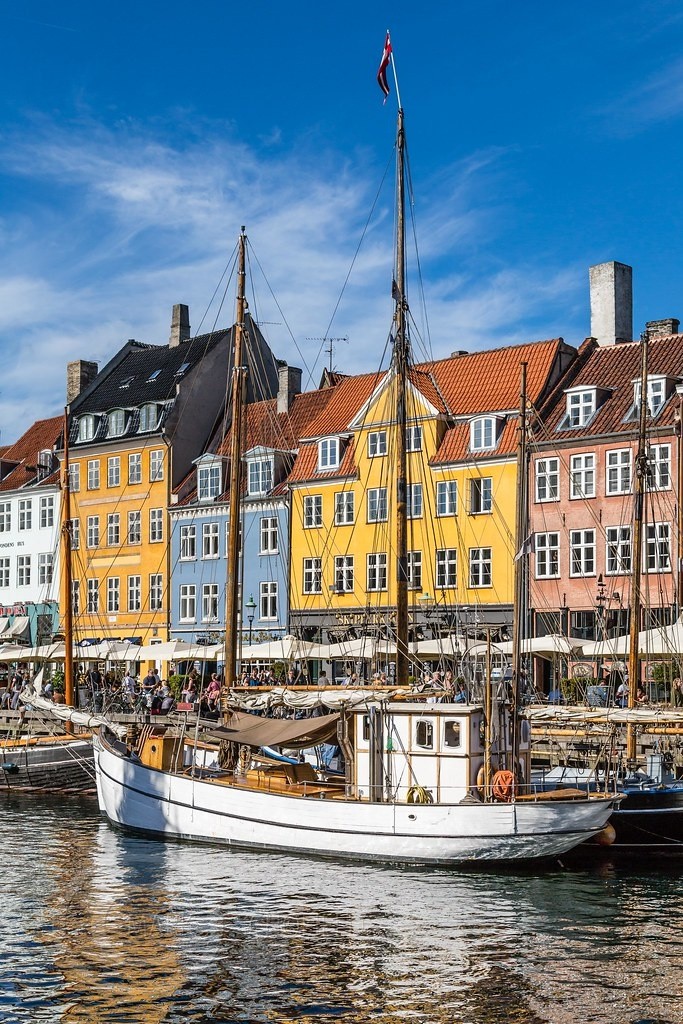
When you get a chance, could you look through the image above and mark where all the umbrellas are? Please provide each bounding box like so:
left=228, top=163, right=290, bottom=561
left=0, top=640, right=81, bottom=667
left=108, top=638, right=204, bottom=675
left=581, top=618, right=683, bottom=656
left=51, top=640, right=142, bottom=673
left=372, top=634, right=496, bottom=674
left=0, top=642, right=28, bottom=653
left=173, top=640, right=251, bottom=675
left=293, top=635, right=395, bottom=674
left=216, top=634, right=329, bottom=673
left=490, top=632, right=599, bottom=703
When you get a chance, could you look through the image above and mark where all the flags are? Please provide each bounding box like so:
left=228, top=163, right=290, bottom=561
left=376, top=31, right=391, bottom=105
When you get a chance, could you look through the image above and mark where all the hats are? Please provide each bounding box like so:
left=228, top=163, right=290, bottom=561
left=624, top=674, right=630, bottom=683
left=372, top=673, right=380, bottom=678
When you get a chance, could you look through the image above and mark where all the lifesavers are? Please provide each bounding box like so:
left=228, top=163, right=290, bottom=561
left=476, top=765, right=498, bottom=799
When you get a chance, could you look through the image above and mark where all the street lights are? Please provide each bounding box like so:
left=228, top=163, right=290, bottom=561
left=244, top=592, right=257, bottom=678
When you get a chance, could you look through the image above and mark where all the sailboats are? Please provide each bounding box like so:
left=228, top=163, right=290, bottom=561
left=1, top=24, right=683, bottom=863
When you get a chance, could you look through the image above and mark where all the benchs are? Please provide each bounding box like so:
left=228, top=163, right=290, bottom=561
left=516, top=788, right=586, bottom=801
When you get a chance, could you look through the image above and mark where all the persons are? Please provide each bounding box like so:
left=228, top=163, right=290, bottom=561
left=599, top=680, right=616, bottom=707
left=428, top=671, right=471, bottom=703
left=371, top=673, right=387, bottom=686
left=317, top=671, right=330, bottom=715
left=84, top=668, right=174, bottom=715
left=535, top=685, right=563, bottom=705
left=616, top=675, right=648, bottom=709
left=0, top=661, right=66, bottom=709
left=203, top=672, right=220, bottom=712
left=184, top=668, right=202, bottom=703
left=239, top=667, right=308, bottom=720
left=673, top=678, right=683, bottom=707
